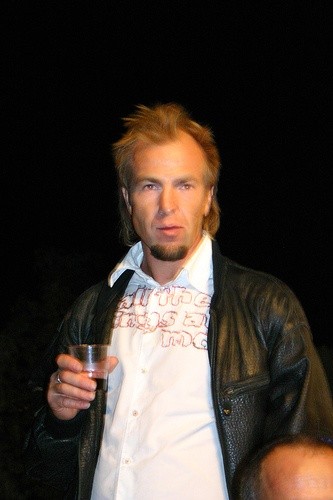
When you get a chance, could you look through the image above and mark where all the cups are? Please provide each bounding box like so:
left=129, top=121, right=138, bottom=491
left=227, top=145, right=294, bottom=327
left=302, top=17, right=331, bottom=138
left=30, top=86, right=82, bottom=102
left=69, top=345, right=109, bottom=392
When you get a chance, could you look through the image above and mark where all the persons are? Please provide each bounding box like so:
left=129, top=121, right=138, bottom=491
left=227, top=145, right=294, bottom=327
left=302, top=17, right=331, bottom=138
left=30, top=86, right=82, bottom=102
left=231, top=431, right=333, bottom=499
left=0, top=105, right=333, bottom=499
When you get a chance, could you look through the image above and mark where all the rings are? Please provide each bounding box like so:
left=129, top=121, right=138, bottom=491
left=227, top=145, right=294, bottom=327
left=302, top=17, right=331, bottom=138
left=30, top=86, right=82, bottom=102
left=55, top=371, right=62, bottom=384
